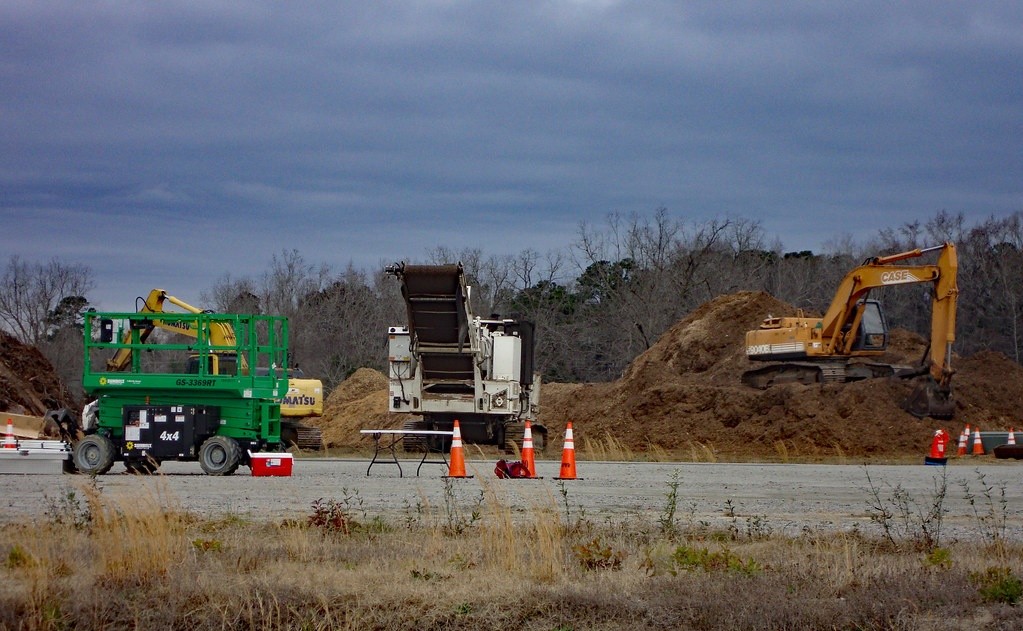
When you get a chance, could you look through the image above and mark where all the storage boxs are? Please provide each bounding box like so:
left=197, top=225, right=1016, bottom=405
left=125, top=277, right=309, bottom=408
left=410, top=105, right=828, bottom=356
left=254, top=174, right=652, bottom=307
left=251, top=452, right=294, bottom=477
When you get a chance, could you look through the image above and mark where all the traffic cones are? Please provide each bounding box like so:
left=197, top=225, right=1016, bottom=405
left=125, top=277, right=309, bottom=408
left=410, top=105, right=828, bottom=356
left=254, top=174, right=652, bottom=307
left=440, top=420, right=474, bottom=478
left=957, top=431, right=966, bottom=456
left=552, top=422, right=583, bottom=480
left=965, top=424, right=970, bottom=444
left=523, top=421, right=543, bottom=479
left=1008, top=427, right=1015, bottom=445
left=970, top=424, right=984, bottom=455
left=4, top=419, right=17, bottom=448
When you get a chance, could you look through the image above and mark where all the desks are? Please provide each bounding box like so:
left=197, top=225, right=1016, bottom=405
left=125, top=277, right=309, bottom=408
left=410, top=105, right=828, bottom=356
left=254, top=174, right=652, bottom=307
left=359, top=429, right=453, bottom=479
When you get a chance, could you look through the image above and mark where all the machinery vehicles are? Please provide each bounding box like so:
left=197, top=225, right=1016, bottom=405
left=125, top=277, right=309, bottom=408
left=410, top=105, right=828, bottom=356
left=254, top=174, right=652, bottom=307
left=106, top=289, right=323, bottom=451
left=384, top=259, right=543, bottom=455
left=741, top=238, right=959, bottom=420
